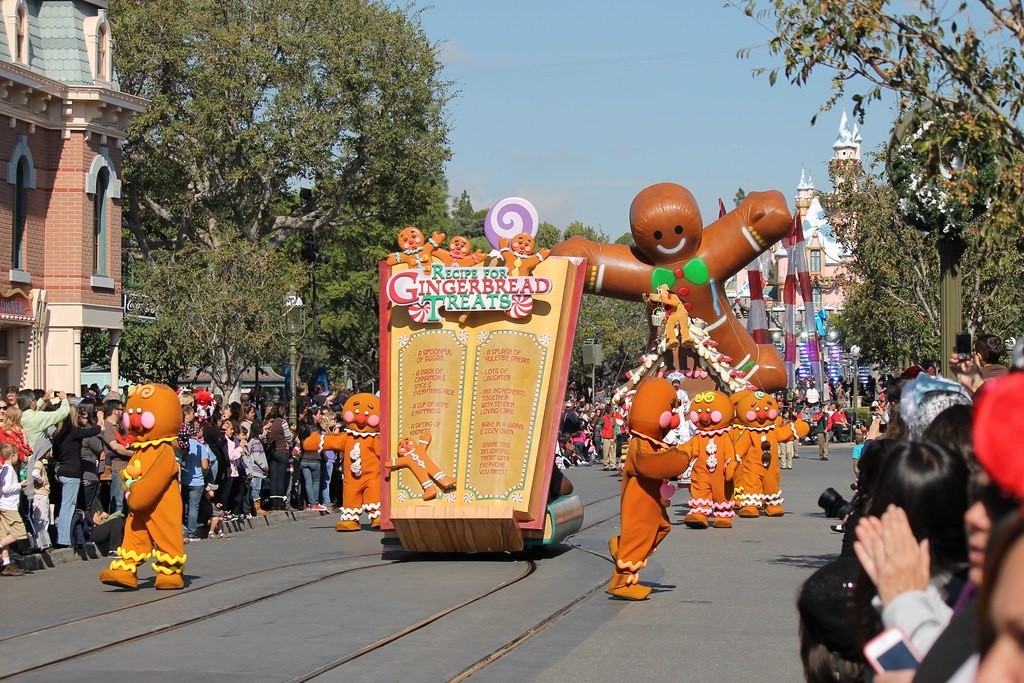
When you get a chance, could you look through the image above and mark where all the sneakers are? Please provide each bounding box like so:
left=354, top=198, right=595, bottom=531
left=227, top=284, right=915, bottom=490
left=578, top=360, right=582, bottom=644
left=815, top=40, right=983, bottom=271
left=1, top=563, right=24, bottom=575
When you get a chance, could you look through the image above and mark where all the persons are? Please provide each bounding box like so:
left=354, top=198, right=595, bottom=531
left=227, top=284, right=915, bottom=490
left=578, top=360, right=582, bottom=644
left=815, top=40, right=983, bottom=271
left=555, top=372, right=695, bottom=471
left=777, top=334, right=1024, bottom=683
left=0, top=381, right=376, bottom=574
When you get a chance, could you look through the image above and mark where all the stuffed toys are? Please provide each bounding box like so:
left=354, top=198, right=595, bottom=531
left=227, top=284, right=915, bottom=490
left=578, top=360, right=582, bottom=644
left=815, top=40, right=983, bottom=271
left=100, top=384, right=187, bottom=590
left=607, top=377, right=810, bottom=599
left=303, top=393, right=381, bottom=531
left=641, top=284, right=695, bottom=349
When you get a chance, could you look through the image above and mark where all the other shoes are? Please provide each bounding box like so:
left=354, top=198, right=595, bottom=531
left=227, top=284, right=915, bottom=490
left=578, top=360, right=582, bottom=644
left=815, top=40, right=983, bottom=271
left=108, top=552, right=122, bottom=558
left=222, top=512, right=252, bottom=522
left=612, top=468, right=617, bottom=471
left=822, top=458, right=828, bottom=461
left=604, top=468, right=609, bottom=471
left=308, top=504, right=327, bottom=511
left=188, top=536, right=201, bottom=542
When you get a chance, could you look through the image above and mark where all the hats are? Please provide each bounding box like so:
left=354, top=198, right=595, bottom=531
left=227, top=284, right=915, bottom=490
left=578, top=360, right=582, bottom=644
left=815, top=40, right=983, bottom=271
left=973, top=373, right=1024, bottom=499
left=836, top=404, right=842, bottom=411
left=667, top=372, right=685, bottom=384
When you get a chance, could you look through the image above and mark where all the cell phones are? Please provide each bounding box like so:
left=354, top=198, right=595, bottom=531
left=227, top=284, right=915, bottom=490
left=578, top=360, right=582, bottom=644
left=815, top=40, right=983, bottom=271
left=870, top=406, right=876, bottom=412
left=93, top=411, right=98, bottom=417
left=879, top=424, right=887, bottom=433
left=54, top=392, right=59, bottom=397
left=863, top=624, right=924, bottom=674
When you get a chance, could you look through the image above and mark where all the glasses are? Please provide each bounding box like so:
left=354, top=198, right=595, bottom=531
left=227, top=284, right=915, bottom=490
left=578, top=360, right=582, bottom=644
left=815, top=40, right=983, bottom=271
left=115, top=407, right=124, bottom=412
left=966, top=465, right=1018, bottom=522
left=0, top=406, right=7, bottom=410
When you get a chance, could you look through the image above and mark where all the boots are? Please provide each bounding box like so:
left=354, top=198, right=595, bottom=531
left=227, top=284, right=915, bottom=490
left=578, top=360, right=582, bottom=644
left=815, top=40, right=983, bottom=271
left=270, top=495, right=289, bottom=513
left=254, top=500, right=269, bottom=516
left=100, top=465, right=112, bottom=480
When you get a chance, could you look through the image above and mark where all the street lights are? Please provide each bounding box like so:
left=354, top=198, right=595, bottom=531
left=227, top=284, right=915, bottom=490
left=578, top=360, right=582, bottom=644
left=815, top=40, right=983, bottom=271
left=280, top=284, right=305, bottom=425
left=851, top=345, right=861, bottom=421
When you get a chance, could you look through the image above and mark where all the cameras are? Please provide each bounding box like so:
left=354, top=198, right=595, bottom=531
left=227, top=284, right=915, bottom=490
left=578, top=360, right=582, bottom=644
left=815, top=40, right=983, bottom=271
left=956, top=333, right=971, bottom=361
left=819, top=487, right=858, bottom=523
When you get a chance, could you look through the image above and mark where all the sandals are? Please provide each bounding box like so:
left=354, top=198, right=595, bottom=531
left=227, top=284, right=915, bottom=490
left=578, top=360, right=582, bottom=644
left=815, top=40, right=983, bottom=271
left=217, top=532, right=230, bottom=539
left=208, top=532, right=220, bottom=540
